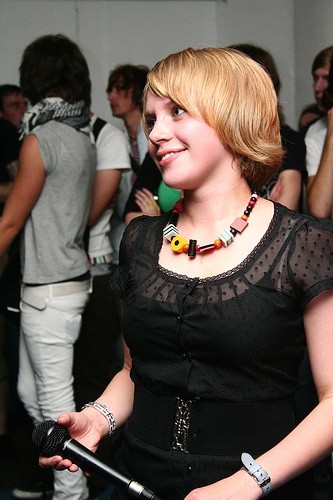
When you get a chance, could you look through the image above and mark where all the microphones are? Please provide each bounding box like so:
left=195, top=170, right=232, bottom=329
left=31, top=420, right=160, bottom=500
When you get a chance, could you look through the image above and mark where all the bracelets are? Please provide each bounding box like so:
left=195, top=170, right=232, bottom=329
left=83, top=402, right=116, bottom=435
left=240, top=451, right=273, bottom=494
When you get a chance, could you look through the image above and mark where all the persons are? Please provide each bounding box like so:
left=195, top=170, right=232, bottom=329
left=0, top=34, right=96, bottom=500
left=304, top=67, right=333, bottom=218
left=299, top=46, right=333, bottom=135
left=106, top=64, right=185, bottom=274
left=227, top=44, right=309, bottom=215
left=31, top=47, right=333, bottom=500
left=74, top=112, right=137, bottom=412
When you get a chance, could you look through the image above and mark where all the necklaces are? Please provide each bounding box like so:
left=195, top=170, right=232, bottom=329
left=163, top=188, right=259, bottom=256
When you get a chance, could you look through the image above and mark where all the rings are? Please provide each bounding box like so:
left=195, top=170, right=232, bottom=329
left=146, top=200, right=152, bottom=207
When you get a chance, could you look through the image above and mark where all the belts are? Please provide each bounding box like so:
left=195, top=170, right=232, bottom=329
left=20, top=279, right=90, bottom=310
